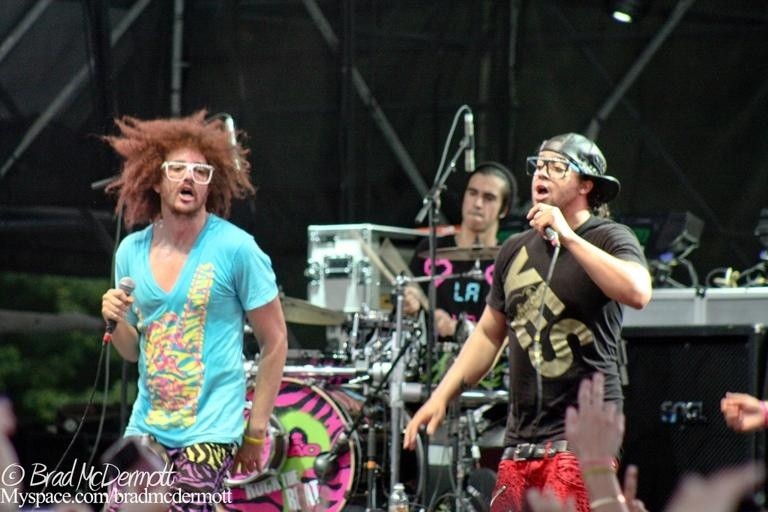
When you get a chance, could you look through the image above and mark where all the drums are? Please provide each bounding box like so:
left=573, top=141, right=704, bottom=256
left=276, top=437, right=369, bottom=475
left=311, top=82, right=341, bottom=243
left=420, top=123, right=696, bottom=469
left=214, top=375, right=424, bottom=512
left=325, top=307, right=422, bottom=380
left=220, top=399, right=286, bottom=485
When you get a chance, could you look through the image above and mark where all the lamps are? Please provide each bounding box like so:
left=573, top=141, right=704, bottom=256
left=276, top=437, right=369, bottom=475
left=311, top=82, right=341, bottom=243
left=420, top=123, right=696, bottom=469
left=610, top=1, right=639, bottom=26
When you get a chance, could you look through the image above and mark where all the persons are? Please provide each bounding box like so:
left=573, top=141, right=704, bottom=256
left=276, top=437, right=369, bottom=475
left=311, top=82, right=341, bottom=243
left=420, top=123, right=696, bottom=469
left=400, top=134, right=653, bottom=511
left=0, top=392, right=177, bottom=512
left=392, top=160, right=518, bottom=511
left=96, top=105, right=290, bottom=512
left=520, top=369, right=766, bottom=511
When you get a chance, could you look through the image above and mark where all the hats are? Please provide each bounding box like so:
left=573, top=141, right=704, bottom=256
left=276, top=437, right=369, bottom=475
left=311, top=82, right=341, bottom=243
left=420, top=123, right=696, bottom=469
left=470, top=161, right=516, bottom=219
left=541, top=131, right=621, bottom=205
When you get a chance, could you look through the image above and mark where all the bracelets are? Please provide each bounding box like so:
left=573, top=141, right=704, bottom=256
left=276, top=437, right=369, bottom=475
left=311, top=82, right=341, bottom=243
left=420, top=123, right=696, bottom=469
left=241, top=428, right=270, bottom=448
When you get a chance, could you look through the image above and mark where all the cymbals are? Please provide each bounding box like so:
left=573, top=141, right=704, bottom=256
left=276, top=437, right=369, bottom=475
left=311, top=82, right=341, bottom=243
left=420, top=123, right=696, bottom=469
left=279, top=292, right=348, bottom=325
left=416, top=245, right=505, bottom=261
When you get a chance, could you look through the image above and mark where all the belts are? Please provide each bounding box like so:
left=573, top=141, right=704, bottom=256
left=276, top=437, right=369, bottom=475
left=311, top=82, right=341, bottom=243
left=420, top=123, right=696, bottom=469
left=503, top=440, right=573, bottom=462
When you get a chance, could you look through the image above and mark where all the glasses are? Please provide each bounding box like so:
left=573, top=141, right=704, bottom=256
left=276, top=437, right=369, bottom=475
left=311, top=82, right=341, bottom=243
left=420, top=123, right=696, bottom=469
left=526, top=157, right=580, bottom=181
left=159, top=161, right=215, bottom=184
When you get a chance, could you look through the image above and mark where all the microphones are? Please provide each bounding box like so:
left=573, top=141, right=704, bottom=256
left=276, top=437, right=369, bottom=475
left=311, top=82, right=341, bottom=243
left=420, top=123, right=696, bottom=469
left=101, top=276, right=135, bottom=347
left=533, top=209, right=559, bottom=247
left=463, top=113, right=476, bottom=173
left=224, top=117, right=240, bottom=170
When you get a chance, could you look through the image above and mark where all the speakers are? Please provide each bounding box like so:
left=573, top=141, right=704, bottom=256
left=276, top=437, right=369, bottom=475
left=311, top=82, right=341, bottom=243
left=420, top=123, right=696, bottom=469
left=618, top=321, right=768, bottom=512
